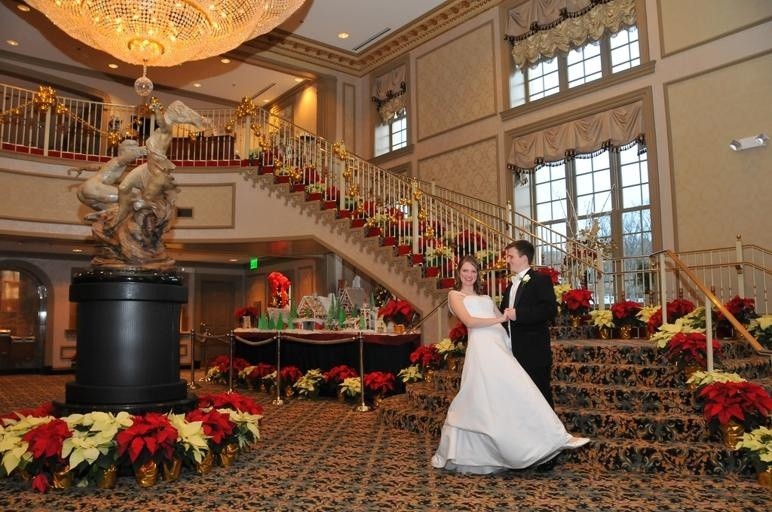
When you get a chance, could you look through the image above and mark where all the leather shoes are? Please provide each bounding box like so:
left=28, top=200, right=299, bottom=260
left=559, top=435, right=591, bottom=449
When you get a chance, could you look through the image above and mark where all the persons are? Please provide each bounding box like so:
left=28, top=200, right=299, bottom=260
left=430, top=254, right=592, bottom=476
left=106, top=99, right=205, bottom=233
left=77, top=139, right=150, bottom=208
left=499, top=240, right=562, bottom=474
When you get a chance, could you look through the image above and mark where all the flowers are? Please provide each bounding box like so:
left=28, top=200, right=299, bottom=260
left=635, top=294, right=772, bottom=476
left=210, top=271, right=468, bottom=401
left=537, top=266, right=639, bottom=340
left=0, top=391, right=265, bottom=493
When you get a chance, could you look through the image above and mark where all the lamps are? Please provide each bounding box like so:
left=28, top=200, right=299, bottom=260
left=128, top=37, right=165, bottom=98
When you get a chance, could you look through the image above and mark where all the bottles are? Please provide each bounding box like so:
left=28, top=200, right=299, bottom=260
left=258, top=305, right=404, bottom=333
